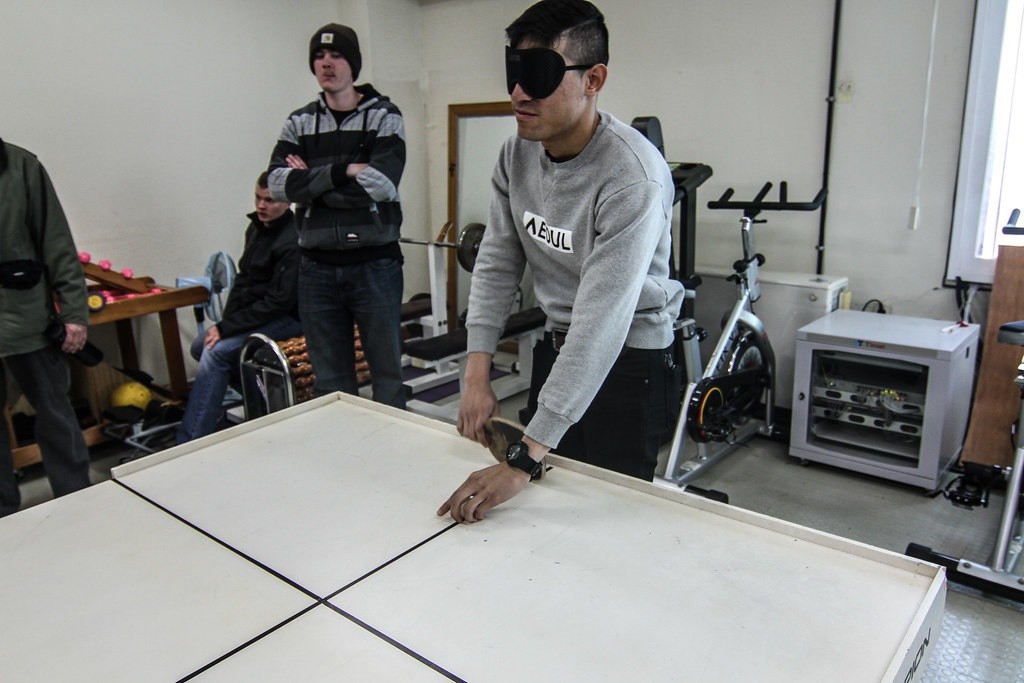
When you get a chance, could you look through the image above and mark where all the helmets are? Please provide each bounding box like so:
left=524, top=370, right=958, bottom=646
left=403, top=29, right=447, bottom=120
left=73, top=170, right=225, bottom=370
left=111, top=379, right=151, bottom=411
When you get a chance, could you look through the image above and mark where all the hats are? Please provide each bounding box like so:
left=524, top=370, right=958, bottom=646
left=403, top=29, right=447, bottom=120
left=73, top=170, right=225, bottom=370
left=308, top=23, right=361, bottom=82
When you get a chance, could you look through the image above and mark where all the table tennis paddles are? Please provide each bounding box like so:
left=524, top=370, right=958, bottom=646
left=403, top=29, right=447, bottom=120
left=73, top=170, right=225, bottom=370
left=480, top=415, right=547, bottom=481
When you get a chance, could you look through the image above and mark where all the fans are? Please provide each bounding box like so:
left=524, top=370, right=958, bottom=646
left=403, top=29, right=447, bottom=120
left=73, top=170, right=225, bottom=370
left=175, top=250, right=243, bottom=406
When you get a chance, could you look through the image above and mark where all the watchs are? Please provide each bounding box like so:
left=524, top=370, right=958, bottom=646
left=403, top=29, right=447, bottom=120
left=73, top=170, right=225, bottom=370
left=505, top=440, right=553, bottom=482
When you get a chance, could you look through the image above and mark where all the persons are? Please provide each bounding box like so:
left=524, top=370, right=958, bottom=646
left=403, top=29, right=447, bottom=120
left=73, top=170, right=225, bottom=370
left=0, top=138, right=90, bottom=515
left=176, top=172, right=304, bottom=448
left=266, top=23, right=407, bottom=409
left=435, top=0, right=684, bottom=524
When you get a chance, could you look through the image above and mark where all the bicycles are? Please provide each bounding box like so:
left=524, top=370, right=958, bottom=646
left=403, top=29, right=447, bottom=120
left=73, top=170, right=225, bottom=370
left=645, top=184, right=834, bottom=506
left=906, top=209, right=1024, bottom=602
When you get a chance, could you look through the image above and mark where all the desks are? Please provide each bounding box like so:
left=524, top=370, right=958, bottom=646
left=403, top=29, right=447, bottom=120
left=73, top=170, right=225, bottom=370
left=0, top=392, right=945, bottom=683
